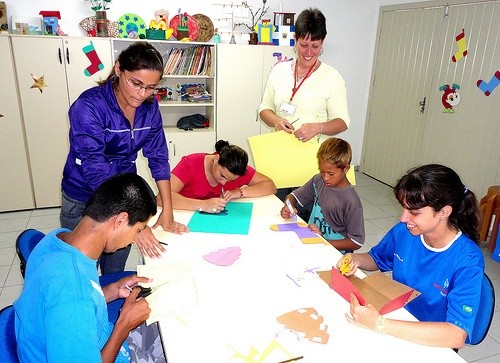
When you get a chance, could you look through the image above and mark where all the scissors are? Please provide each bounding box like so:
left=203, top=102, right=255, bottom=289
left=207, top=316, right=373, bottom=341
left=339, top=257, right=354, bottom=275
left=130, top=285, right=155, bottom=299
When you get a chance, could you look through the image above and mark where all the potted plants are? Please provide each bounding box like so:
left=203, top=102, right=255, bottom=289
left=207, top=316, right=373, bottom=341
left=235, top=0, right=270, bottom=44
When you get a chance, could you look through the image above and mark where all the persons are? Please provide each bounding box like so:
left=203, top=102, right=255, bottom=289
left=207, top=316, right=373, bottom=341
left=156, top=140, right=277, bottom=214
left=11, top=172, right=158, bottom=363
left=280, top=138, right=365, bottom=256
left=258, top=7, right=350, bottom=224
left=334, top=163, right=486, bottom=349
left=58, top=40, right=189, bottom=277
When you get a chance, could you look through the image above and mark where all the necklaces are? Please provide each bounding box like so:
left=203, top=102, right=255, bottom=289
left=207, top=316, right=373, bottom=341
left=297, top=63, right=307, bottom=82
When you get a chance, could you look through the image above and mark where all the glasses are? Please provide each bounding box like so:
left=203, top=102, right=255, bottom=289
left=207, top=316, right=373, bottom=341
left=123, top=71, right=160, bottom=95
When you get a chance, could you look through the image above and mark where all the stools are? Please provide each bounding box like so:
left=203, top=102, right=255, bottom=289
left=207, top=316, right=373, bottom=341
left=480, top=186, right=500, bottom=254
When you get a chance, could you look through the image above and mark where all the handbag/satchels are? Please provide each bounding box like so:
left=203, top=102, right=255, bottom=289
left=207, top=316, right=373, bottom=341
left=177, top=113, right=206, bottom=131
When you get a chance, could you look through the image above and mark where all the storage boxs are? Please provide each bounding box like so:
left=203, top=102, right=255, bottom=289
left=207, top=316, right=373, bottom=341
left=146, top=29, right=165, bottom=40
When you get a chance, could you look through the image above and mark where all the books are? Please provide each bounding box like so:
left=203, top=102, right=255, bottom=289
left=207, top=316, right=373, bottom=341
left=163, top=45, right=212, bottom=75
left=184, top=84, right=212, bottom=103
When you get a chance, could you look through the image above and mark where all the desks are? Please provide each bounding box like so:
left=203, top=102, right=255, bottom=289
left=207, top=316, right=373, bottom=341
left=141, top=195, right=468, bottom=363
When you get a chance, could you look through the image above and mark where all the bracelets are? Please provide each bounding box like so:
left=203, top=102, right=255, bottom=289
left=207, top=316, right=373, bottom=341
left=377, top=314, right=386, bottom=334
left=317, top=123, right=322, bottom=136
left=236, top=189, right=243, bottom=198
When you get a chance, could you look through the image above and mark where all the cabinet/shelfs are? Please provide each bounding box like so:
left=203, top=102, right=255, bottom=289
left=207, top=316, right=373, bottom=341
left=10, top=33, right=112, bottom=209
left=112, top=38, right=216, bottom=198
left=0, top=34, right=35, bottom=214
left=206, top=42, right=299, bottom=173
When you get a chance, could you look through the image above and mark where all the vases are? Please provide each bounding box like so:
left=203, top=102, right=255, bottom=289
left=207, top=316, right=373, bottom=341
left=95, top=11, right=109, bottom=37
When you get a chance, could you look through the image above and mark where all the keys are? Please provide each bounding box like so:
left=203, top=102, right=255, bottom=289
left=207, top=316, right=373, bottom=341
left=317, top=137, right=320, bottom=143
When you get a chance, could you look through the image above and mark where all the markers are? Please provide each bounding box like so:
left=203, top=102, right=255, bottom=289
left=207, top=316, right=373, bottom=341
left=285, top=198, right=297, bottom=221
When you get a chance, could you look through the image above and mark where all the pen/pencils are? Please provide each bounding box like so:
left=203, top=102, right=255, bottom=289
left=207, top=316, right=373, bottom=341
left=291, top=118, right=300, bottom=124
left=279, top=356, right=303, bottom=363
left=222, top=187, right=225, bottom=199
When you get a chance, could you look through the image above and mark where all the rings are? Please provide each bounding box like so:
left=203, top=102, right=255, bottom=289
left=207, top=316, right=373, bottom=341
left=300, top=136, right=304, bottom=140
left=213, top=208, right=217, bottom=212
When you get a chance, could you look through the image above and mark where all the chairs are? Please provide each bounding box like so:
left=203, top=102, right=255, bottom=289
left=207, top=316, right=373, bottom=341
left=0, top=305, right=21, bottom=363
left=16, top=228, right=137, bottom=326
left=453, top=272, right=496, bottom=353
